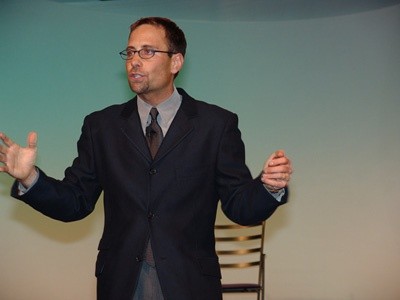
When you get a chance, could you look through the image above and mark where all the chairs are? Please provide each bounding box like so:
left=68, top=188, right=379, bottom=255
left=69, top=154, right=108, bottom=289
left=214, top=222, right=266, bottom=300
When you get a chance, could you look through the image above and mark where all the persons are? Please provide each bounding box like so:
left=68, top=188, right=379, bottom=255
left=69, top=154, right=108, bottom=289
left=0, top=17, right=294, bottom=300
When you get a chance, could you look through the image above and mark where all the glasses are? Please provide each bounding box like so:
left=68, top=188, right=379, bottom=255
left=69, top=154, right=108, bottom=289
left=120, top=49, right=176, bottom=60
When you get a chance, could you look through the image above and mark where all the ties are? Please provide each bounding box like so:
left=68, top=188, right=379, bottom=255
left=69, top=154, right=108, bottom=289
left=146, top=107, right=163, bottom=267
